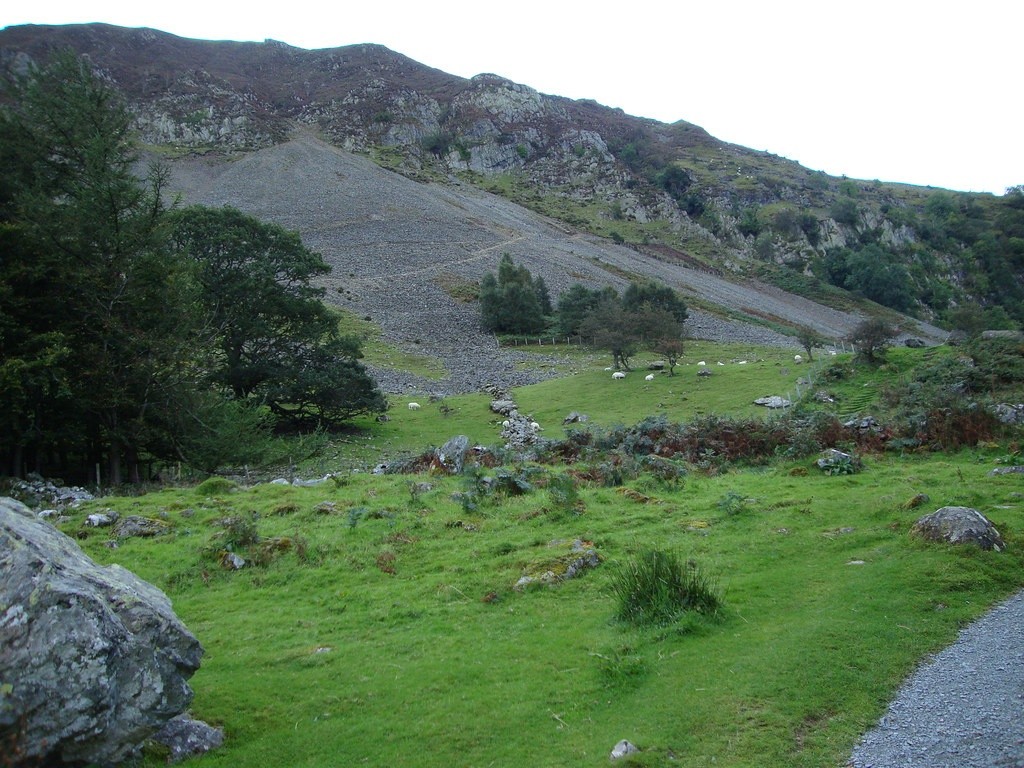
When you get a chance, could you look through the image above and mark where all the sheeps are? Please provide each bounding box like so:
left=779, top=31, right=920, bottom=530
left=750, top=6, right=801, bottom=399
left=503, top=421, right=540, bottom=430
left=409, top=402, right=421, bottom=411
left=604, top=351, right=837, bottom=381
